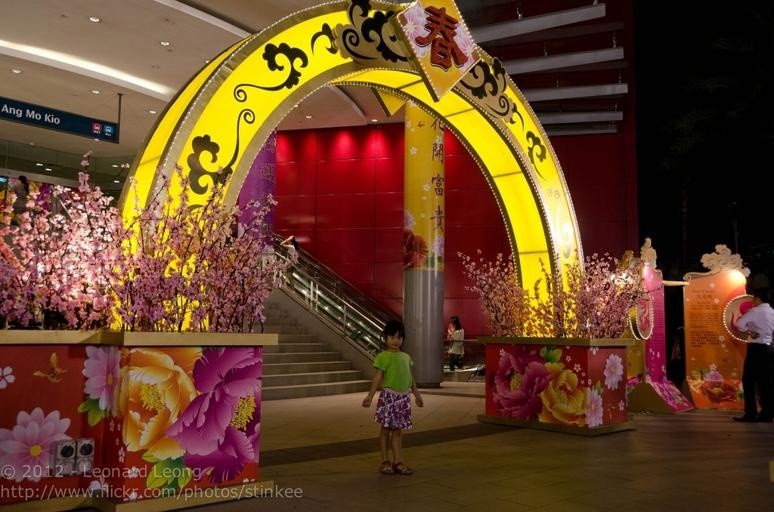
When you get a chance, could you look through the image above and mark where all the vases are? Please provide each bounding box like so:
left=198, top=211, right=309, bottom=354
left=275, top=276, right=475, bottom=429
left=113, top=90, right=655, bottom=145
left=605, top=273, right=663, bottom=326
left=1, top=329, right=278, bottom=512
left=476, top=337, right=643, bottom=438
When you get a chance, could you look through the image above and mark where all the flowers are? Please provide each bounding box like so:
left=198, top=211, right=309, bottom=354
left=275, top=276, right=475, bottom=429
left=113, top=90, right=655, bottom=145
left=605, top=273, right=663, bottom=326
left=456, top=249, right=665, bottom=338
left=1, top=147, right=300, bottom=332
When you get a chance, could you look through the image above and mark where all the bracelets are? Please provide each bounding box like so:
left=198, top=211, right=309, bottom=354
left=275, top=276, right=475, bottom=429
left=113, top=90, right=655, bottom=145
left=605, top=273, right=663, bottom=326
left=286, top=239, right=288, bottom=242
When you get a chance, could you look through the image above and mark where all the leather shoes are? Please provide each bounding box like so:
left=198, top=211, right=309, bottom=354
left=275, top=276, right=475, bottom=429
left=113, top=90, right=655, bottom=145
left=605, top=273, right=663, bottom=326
left=731, top=411, right=774, bottom=423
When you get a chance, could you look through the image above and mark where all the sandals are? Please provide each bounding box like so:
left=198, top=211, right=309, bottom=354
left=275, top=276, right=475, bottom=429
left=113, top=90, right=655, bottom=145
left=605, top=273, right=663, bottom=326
left=379, top=460, right=412, bottom=476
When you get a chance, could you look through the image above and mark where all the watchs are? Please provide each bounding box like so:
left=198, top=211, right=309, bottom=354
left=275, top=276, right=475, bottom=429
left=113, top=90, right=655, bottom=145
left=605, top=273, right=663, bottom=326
left=746, top=329, right=753, bottom=336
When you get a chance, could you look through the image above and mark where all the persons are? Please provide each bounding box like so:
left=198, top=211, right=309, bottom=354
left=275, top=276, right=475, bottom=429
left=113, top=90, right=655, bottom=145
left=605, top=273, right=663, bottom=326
left=280, top=234, right=300, bottom=289
left=731, top=287, right=774, bottom=423
left=445, top=315, right=467, bottom=372
left=360, top=318, right=426, bottom=477
left=9, top=175, right=31, bottom=225
